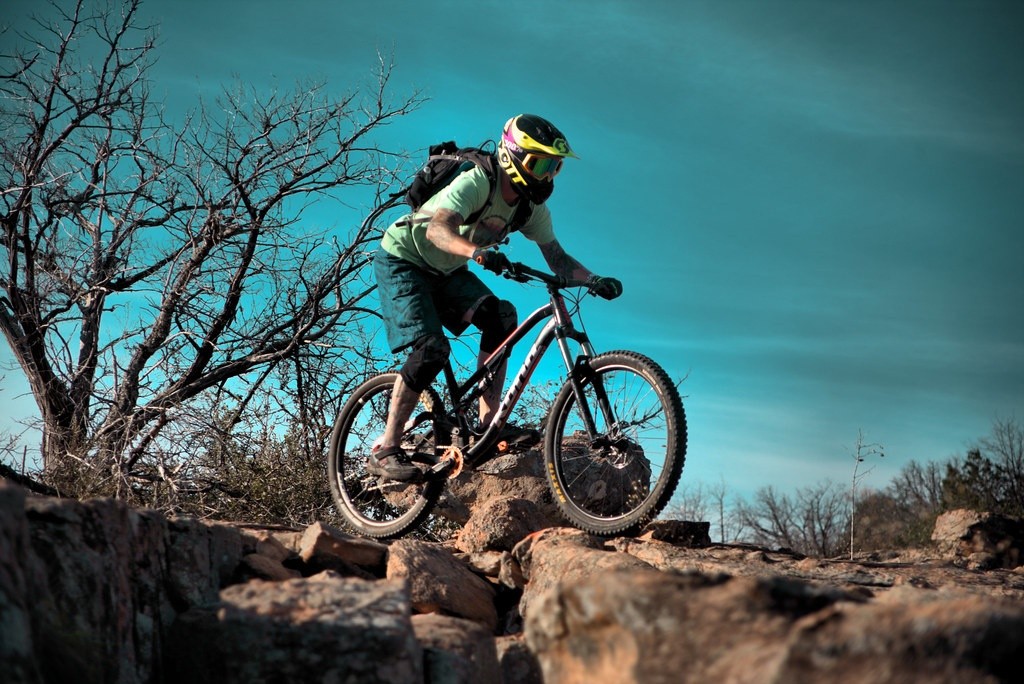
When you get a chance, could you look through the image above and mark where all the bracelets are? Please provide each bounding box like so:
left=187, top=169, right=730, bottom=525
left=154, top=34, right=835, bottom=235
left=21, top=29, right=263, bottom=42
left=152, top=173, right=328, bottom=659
left=586, top=273, right=596, bottom=283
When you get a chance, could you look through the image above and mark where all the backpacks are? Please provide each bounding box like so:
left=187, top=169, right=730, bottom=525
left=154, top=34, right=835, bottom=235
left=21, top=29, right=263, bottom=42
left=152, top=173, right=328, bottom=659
left=405, top=140, right=534, bottom=232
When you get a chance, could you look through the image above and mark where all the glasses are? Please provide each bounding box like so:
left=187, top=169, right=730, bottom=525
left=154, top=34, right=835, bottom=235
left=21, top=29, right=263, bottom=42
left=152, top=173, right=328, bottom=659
left=520, top=153, right=563, bottom=181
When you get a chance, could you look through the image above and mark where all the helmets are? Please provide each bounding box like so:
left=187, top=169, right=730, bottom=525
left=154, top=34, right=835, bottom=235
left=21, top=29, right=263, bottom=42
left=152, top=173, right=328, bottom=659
left=497, top=114, right=580, bottom=204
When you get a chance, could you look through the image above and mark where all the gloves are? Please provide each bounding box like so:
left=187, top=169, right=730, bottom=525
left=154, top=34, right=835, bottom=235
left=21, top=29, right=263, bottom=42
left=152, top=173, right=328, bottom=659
left=588, top=276, right=623, bottom=300
left=477, top=250, right=516, bottom=277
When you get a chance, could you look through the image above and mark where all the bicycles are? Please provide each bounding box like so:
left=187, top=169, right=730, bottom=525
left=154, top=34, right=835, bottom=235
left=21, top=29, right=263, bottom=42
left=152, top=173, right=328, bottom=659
left=325, top=252, right=688, bottom=542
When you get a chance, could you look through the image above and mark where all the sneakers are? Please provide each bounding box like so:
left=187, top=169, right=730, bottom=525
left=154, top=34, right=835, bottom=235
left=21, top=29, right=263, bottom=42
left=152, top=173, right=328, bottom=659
left=473, top=421, right=542, bottom=453
left=365, top=447, right=422, bottom=482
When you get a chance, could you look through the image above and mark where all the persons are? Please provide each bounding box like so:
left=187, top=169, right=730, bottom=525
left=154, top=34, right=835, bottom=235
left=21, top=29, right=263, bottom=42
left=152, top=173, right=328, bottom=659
left=366, top=114, right=622, bottom=482
